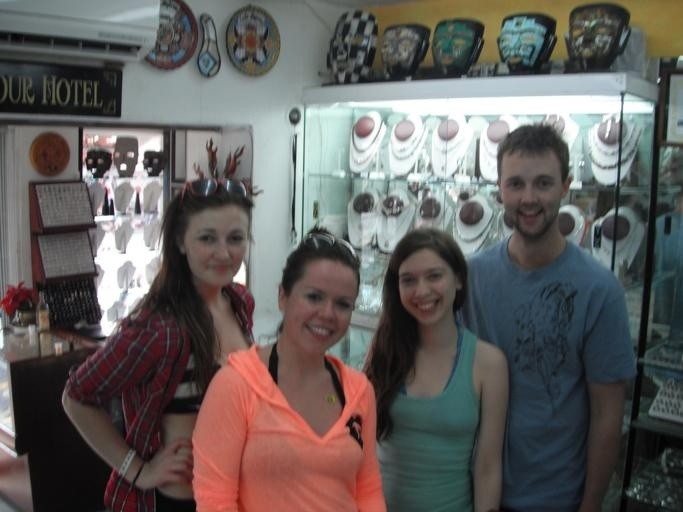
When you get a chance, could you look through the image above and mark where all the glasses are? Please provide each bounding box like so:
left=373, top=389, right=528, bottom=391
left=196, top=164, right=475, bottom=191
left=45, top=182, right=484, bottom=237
left=177, top=179, right=247, bottom=209
left=304, top=233, right=356, bottom=260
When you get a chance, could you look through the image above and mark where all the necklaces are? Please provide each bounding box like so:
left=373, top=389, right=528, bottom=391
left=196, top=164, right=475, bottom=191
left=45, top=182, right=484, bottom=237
left=275, top=352, right=337, bottom=406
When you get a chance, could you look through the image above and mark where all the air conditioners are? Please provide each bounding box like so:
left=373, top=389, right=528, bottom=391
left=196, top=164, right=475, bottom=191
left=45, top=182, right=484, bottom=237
left=0, top=0, right=160, bottom=72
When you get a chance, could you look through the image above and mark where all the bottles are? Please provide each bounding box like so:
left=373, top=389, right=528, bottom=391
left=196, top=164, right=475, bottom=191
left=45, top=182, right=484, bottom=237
left=35, top=290, right=50, bottom=334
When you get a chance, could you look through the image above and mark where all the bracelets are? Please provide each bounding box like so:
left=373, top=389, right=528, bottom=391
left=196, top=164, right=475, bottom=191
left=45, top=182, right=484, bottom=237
left=131, top=458, right=146, bottom=487
left=116, top=448, right=136, bottom=480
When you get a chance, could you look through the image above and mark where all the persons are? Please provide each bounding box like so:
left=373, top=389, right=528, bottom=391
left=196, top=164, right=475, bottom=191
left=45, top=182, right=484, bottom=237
left=451, top=123, right=637, bottom=512
left=190, top=218, right=386, bottom=512
left=59, top=177, right=256, bottom=512
left=360, top=228, right=508, bottom=512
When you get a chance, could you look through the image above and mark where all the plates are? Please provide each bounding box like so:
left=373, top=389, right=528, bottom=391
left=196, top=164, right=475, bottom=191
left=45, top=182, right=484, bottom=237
left=225, top=6, right=281, bottom=78
left=143, top=1, right=198, bottom=71
